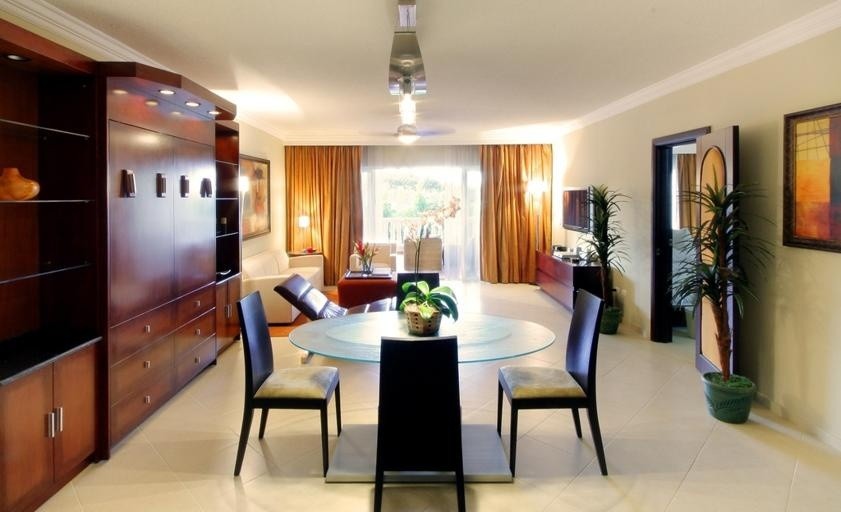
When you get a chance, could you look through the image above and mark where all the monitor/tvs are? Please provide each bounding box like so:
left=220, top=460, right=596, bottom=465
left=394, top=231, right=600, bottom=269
left=563, top=186, right=590, bottom=232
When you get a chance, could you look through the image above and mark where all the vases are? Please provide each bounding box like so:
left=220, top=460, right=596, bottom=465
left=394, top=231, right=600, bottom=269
left=363, top=263, right=373, bottom=274
left=404, top=305, right=442, bottom=338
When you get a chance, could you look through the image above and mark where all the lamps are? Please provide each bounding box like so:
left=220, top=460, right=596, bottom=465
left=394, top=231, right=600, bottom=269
left=532, top=191, right=544, bottom=250
left=298, top=215, right=310, bottom=253
left=385, top=0, right=429, bottom=146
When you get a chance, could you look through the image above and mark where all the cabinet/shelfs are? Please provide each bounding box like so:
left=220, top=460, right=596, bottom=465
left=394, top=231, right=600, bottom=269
left=215, top=111, right=243, bottom=356
left=0, top=16, right=106, bottom=512
left=106, top=59, right=217, bottom=459
left=534, top=248, right=613, bottom=315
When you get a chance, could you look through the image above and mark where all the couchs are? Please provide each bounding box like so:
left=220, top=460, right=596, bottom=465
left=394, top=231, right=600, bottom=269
left=403, top=238, right=443, bottom=271
left=349, top=244, right=391, bottom=270
left=242, top=246, right=325, bottom=325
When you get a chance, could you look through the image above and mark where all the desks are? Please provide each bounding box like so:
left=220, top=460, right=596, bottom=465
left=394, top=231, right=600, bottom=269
left=289, top=306, right=556, bottom=483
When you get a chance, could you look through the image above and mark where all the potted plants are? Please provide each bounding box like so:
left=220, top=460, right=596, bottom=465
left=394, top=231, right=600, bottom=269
left=575, top=185, right=633, bottom=335
left=667, top=161, right=780, bottom=425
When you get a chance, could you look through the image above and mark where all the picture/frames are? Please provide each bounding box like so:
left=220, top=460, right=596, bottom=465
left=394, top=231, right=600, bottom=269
left=239, top=153, right=271, bottom=241
left=783, top=103, right=840, bottom=253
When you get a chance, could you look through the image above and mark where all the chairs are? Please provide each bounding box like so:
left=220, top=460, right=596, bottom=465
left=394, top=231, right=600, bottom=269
left=373, top=334, right=468, bottom=511
left=233, top=290, right=342, bottom=477
left=498, top=289, right=609, bottom=477
left=274, top=273, right=397, bottom=363
left=396, top=273, right=440, bottom=311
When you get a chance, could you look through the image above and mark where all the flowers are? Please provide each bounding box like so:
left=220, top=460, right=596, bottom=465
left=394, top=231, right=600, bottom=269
left=354, top=240, right=381, bottom=263
left=397, top=195, right=459, bottom=323
